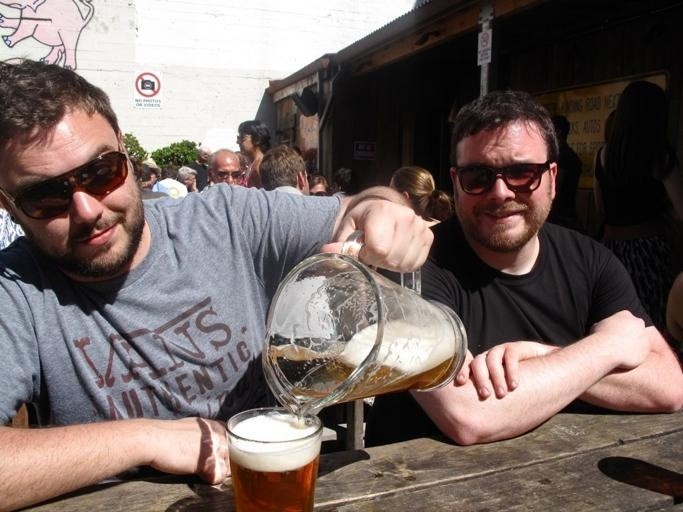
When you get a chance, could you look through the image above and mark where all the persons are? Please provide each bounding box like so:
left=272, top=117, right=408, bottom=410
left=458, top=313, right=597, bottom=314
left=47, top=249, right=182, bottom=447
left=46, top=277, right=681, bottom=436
left=364, top=86, right=682, bottom=448
left=0, top=54, right=436, bottom=512
left=0, top=80, right=682, bottom=360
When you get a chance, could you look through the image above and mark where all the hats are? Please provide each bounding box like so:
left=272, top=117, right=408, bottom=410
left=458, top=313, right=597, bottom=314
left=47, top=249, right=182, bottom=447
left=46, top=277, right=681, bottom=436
left=142, top=157, right=162, bottom=177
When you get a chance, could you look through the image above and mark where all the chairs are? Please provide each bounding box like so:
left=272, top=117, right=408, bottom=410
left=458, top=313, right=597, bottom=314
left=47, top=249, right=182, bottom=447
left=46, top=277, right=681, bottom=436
left=348, top=264, right=422, bottom=451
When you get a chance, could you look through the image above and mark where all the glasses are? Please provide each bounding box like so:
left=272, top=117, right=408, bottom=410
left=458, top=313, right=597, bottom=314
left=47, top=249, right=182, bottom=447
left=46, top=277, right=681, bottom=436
left=1, top=137, right=129, bottom=220
left=455, top=162, right=550, bottom=195
left=309, top=191, right=328, bottom=196
left=214, top=171, right=241, bottom=181
left=237, top=135, right=248, bottom=142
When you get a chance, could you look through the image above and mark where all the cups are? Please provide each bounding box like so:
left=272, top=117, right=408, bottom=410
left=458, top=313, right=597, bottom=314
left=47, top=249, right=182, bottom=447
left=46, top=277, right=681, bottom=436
left=261, top=231, right=467, bottom=420
left=225, top=406, right=325, bottom=512
